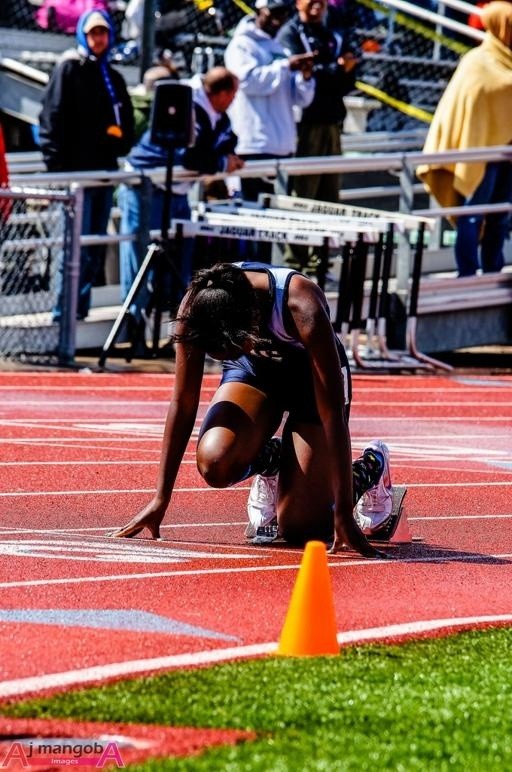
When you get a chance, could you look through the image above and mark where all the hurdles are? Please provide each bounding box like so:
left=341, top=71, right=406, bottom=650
left=95, top=192, right=456, bottom=375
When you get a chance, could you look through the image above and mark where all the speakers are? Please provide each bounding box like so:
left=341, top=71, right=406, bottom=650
left=149, top=82, right=195, bottom=149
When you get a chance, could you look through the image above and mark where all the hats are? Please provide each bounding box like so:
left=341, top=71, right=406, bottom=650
left=82, top=11, right=111, bottom=34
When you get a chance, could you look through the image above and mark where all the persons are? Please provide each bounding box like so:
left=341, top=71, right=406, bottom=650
left=109, top=260, right=401, bottom=560
left=37, top=2, right=360, bottom=357
left=415, top=1, right=512, bottom=275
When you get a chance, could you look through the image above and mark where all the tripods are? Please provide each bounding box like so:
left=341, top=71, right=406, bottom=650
left=97, top=150, right=212, bottom=370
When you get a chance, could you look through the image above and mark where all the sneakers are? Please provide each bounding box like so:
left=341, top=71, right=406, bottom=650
left=355, top=439, right=398, bottom=536
left=246, top=435, right=288, bottom=531
